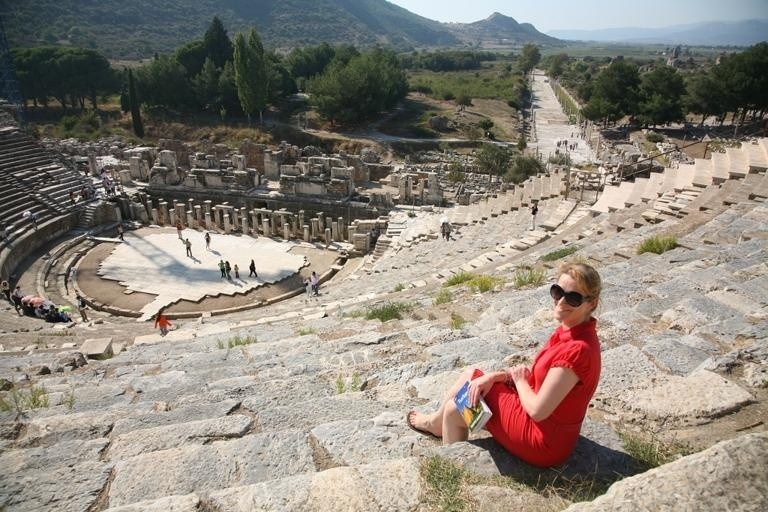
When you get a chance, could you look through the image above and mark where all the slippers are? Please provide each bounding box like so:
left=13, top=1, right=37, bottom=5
left=407, top=411, right=441, bottom=439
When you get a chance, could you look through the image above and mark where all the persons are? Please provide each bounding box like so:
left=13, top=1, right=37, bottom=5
left=555, top=129, right=586, bottom=157
left=234, top=264, right=239, bottom=278
left=1, top=280, right=88, bottom=322
left=407, top=263, right=602, bottom=468
left=371, top=228, right=378, bottom=248
left=176, top=218, right=210, bottom=258
left=217, top=260, right=232, bottom=281
left=528, top=202, right=538, bottom=231
left=248, top=260, right=257, bottom=277
left=69, top=165, right=121, bottom=206
left=303, top=271, right=320, bottom=298
left=31, top=216, right=38, bottom=230
left=117, top=224, right=125, bottom=241
left=155, top=309, right=172, bottom=336
left=440, top=221, right=450, bottom=241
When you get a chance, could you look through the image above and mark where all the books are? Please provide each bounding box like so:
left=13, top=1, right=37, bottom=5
left=453, top=380, right=493, bottom=434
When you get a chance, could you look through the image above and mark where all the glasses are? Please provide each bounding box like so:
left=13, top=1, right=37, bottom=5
left=550, top=284, right=594, bottom=307
